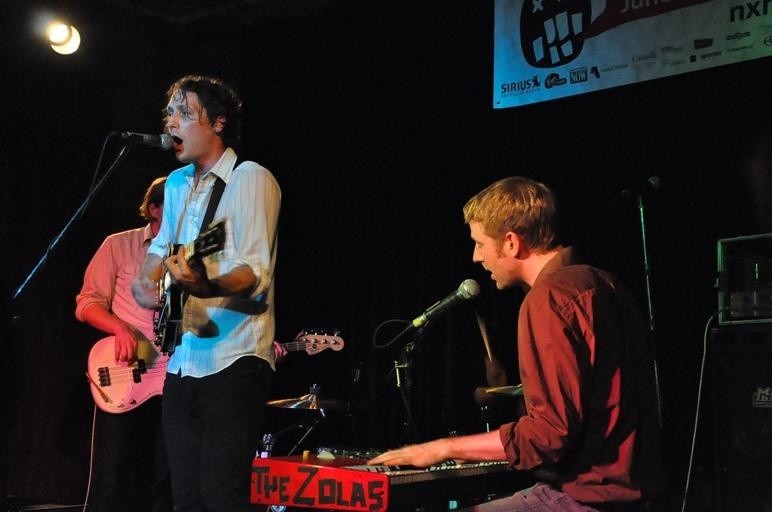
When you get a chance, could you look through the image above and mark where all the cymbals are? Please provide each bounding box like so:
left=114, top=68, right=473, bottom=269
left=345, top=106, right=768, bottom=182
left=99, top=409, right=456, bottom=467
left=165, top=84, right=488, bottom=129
left=485, top=383, right=524, bottom=394
left=264, top=395, right=348, bottom=410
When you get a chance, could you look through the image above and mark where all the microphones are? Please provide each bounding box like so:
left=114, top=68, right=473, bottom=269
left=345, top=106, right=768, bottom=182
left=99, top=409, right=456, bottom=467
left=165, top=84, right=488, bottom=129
left=111, top=126, right=173, bottom=152
left=622, top=173, right=662, bottom=197
left=413, top=278, right=481, bottom=335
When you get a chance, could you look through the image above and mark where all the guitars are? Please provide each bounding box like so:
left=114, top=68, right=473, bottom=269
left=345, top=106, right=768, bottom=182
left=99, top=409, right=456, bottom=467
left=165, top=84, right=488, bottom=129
left=88, top=329, right=345, bottom=416
left=152, top=220, right=233, bottom=356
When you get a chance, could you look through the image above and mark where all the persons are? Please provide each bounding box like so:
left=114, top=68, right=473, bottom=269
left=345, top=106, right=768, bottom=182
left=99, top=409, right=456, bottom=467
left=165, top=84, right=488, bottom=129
left=128, top=75, right=287, bottom=511
left=73, top=176, right=288, bottom=511
left=364, top=175, right=661, bottom=511
